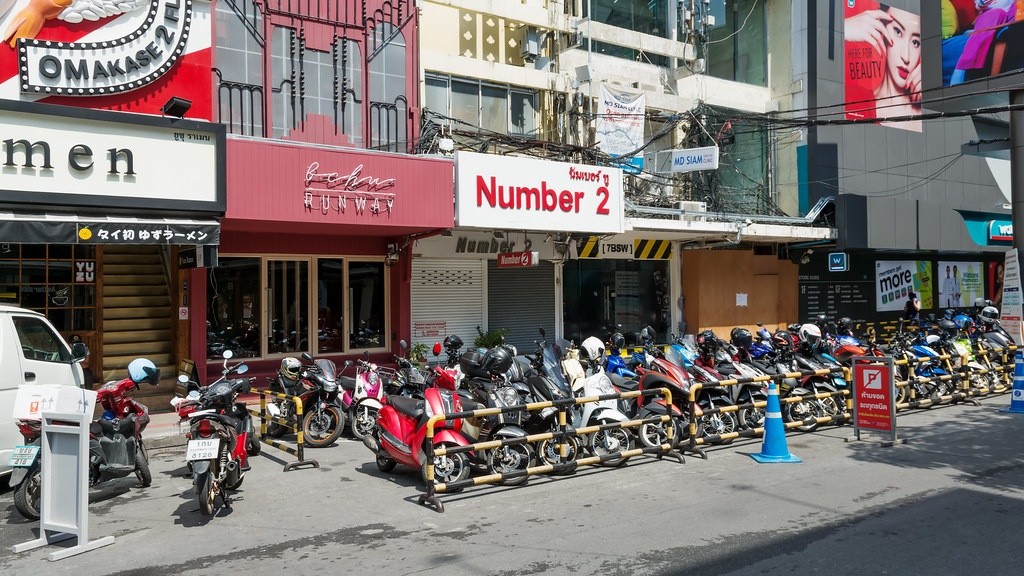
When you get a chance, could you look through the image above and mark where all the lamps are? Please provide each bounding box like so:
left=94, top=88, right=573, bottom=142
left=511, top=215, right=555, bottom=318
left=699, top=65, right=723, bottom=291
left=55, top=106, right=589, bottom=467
left=160, top=97, right=192, bottom=120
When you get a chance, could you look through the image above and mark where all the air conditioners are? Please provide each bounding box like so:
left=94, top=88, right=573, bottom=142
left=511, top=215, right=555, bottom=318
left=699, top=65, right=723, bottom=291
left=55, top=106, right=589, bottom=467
left=672, top=201, right=706, bottom=221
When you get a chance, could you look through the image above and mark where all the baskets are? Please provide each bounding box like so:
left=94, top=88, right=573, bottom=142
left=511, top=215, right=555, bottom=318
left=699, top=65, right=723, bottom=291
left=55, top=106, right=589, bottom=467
left=378, top=365, right=396, bottom=379
left=409, top=368, right=430, bottom=385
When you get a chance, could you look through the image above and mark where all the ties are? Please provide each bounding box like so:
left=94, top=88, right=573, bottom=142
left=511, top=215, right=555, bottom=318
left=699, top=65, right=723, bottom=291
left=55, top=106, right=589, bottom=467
left=955, top=279, right=956, bottom=283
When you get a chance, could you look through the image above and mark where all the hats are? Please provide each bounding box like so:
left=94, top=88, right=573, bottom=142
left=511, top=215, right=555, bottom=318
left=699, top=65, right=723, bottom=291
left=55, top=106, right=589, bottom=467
left=68, top=335, right=82, bottom=343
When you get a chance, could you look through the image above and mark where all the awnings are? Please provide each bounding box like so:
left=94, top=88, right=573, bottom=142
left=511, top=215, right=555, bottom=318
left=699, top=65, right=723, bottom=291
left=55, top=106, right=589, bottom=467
left=0, top=207, right=220, bottom=248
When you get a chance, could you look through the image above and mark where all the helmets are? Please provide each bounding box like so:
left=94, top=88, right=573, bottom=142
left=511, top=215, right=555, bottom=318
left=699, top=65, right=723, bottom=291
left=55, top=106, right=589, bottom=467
left=280, top=357, right=301, bottom=381
left=443, top=335, right=463, bottom=349
left=836, top=317, right=853, bottom=332
left=772, top=330, right=790, bottom=349
left=952, top=315, right=971, bottom=331
left=128, top=358, right=160, bottom=384
left=733, top=328, right=752, bottom=346
left=480, top=347, right=513, bottom=373
left=798, top=324, right=821, bottom=342
left=610, top=332, right=625, bottom=348
left=580, top=336, right=605, bottom=362
left=639, top=326, right=656, bottom=344
left=974, top=298, right=999, bottom=322
left=937, top=320, right=957, bottom=336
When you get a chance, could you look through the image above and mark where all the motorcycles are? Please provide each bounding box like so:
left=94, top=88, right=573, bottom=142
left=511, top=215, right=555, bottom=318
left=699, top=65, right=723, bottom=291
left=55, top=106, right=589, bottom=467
left=206, top=311, right=381, bottom=360
left=169, top=350, right=261, bottom=516
left=8, top=373, right=153, bottom=520
left=266, top=298, right=1015, bottom=493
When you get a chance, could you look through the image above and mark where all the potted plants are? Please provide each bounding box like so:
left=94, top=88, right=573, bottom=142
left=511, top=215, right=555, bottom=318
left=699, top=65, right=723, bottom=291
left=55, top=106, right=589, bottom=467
left=410, top=341, right=431, bottom=372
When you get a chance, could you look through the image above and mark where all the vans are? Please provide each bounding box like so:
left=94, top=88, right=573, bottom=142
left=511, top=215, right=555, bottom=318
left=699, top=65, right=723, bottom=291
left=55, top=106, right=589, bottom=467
left=0, top=303, right=93, bottom=479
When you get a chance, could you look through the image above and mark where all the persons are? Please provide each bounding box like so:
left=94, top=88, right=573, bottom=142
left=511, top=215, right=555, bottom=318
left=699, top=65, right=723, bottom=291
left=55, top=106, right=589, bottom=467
left=903, top=292, right=921, bottom=327
left=69, top=334, right=93, bottom=390
left=844, top=1, right=923, bottom=132
left=242, top=294, right=256, bottom=323
left=219, top=300, right=231, bottom=323
left=940, top=265, right=961, bottom=306
left=942, top=0, right=1018, bottom=88
left=992, top=263, right=1004, bottom=306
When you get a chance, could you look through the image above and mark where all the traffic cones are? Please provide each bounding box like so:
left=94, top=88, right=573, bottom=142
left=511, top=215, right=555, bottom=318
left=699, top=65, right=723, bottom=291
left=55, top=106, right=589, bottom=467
left=748, top=384, right=802, bottom=463
left=1000, top=355, right=1024, bottom=415
